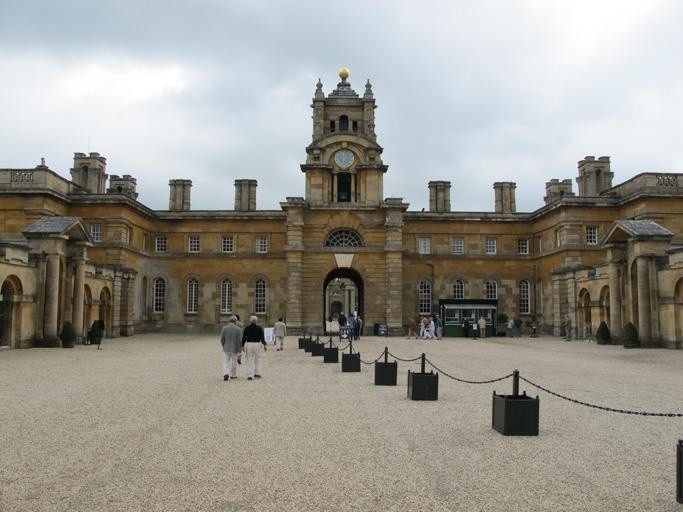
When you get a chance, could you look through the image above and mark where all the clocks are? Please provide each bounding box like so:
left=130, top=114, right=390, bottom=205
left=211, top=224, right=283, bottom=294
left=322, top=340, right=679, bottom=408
left=334, top=150, right=355, bottom=170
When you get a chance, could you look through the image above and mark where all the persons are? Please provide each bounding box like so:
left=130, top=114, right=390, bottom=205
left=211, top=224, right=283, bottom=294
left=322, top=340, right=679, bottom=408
left=338, top=312, right=363, bottom=341
left=274, top=317, right=287, bottom=351
left=464, top=317, right=469, bottom=337
left=507, top=319, right=516, bottom=337
left=478, top=316, right=486, bottom=338
left=406, top=313, right=443, bottom=341
left=240, top=316, right=267, bottom=380
left=560, top=314, right=572, bottom=341
left=235, top=313, right=242, bottom=364
left=220, top=316, right=242, bottom=380
left=514, top=317, right=523, bottom=338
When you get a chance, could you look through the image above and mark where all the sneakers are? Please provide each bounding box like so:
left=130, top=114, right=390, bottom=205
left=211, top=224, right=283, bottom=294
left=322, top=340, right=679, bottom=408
left=223, top=375, right=260, bottom=380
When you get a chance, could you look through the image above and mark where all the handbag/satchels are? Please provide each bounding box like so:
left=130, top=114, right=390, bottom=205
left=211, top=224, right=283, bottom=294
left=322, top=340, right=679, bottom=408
left=237, top=355, right=241, bottom=364
